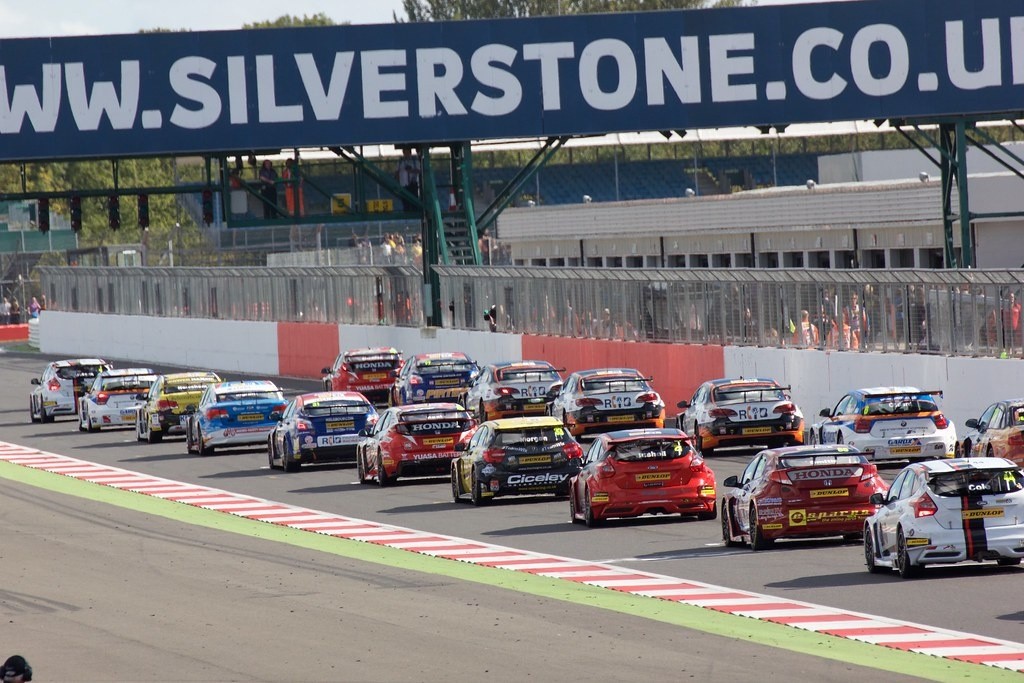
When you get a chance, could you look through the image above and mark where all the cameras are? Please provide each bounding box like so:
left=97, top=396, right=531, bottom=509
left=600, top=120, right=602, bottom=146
left=484, top=310, right=496, bottom=320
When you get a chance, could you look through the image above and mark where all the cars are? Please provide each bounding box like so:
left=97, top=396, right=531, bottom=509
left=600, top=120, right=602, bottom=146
left=675, top=378, right=805, bottom=456
left=863, top=457, right=1024, bottom=578
left=568, top=427, right=717, bottom=528
left=267, top=390, right=380, bottom=472
left=135, top=371, right=224, bottom=445
left=356, top=402, right=479, bottom=488
left=809, top=386, right=957, bottom=463
left=451, top=416, right=585, bottom=506
left=963, top=399, right=1024, bottom=470
left=389, top=351, right=481, bottom=407
left=458, top=359, right=567, bottom=424
left=320, top=347, right=406, bottom=402
left=720, top=444, right=892, bottom=551
left=545, top=367, right=666, bottom=442
left=77, top=367, right=159, bottom=433
left=30, top=358, right=113, bottom=424
left=185, top=380, right=289, bottom=457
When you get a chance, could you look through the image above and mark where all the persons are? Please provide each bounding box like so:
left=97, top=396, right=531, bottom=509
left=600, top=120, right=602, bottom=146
left=1001, top=292, right=1022, bottom=347
left=281, top=158, right=304, bottom=216
left=488, top=305, right=512, bottom=333
left=793, top=291, right=870, bottom=352
left=28, top=294, right=46, bottom=317
left=382, top=232, right=422, bottom=265
left=0, top=655, right=32, bottom=683
left=259, top=160, right=278, bottom=219
left=0, top=297, right=21, bottom=323
left=395, top=148, right=421, bottom=209
left=349, top=233, right=368, bottom=266
left=531, top=295, right=633, bottom=340
